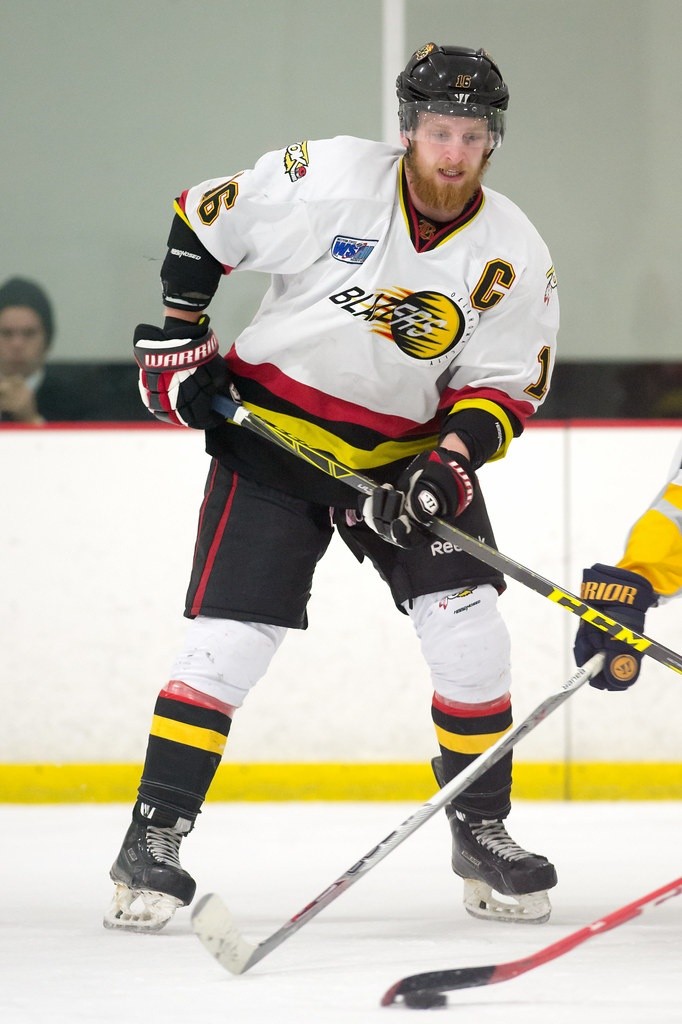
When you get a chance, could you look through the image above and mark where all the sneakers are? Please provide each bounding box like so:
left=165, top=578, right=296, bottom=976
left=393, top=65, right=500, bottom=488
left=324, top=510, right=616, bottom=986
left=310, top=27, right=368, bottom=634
left=447, top=808, right=559, bottom=925
left=102, top=821, right=196, bottom=932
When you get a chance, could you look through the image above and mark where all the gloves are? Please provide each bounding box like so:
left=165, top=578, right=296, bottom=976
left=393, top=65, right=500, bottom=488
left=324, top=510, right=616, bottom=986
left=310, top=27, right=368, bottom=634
left=572, top=563, right=656, bottom=692
left=133, top=314, right=242, bottom=427
left=363, top=447, right=477, bottom=550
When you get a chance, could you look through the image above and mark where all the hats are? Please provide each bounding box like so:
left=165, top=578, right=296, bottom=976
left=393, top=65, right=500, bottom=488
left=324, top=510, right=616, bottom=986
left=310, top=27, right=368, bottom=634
left=1, top=276, right=53, bottom=350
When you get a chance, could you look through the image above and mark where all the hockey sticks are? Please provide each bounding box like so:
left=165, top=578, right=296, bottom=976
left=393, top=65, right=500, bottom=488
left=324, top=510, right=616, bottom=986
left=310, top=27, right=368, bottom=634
left=191, top=391, right=682, bottom=1008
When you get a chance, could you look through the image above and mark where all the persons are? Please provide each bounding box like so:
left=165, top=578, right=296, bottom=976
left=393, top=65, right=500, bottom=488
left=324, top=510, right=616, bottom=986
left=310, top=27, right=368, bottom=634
left=100, top=43, right=558, bottom=935
left=0, top=274, right=97, bottom=426
left=574, top=459, right=682, bottom=693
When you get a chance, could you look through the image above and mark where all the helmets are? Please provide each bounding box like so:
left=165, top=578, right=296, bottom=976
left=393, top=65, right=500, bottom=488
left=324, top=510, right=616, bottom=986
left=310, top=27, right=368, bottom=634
left=394, top=42, right=511, bottom=149
